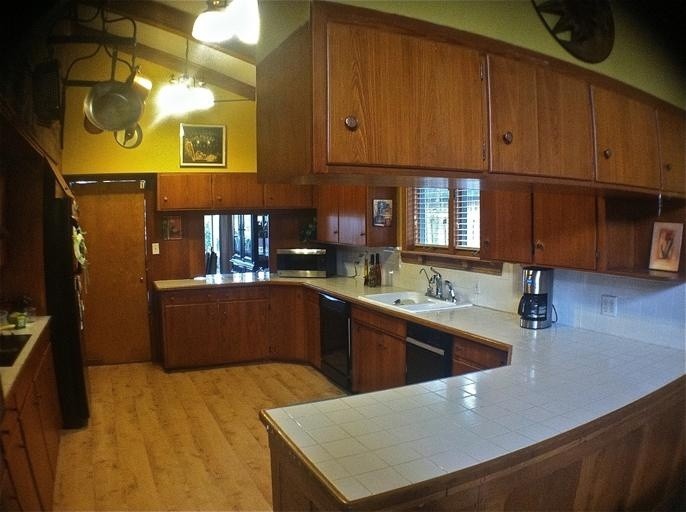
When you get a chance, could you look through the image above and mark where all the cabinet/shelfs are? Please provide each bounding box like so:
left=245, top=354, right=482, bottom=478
left=1, top=324, right=60, bottom=512
left=232, top=214, right=253, bottom=263
left=590, top=84, right=662, bottom=190
left=157, top=173, right=313, bottom=211
left=163, top=286, right=269, bottom=371
left=656, top=107, right=686, bottom=194
left=339, top=186, right=366, bottom=246
left=533, top=192, right=596, bottom=271
left=256, top=215, right=269, bottom=266
left=316, top=186, right=339, bottom=244
left=480, top=190, right=532, bottom=263
left=324, top=16, right=485, bottom=172
left=452, top=338, right=507, bottom=379
left=486, top=51, right=596, bottom=182
left=350, top=303, right=406, bottom=395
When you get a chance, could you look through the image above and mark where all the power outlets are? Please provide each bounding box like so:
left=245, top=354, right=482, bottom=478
left=600, top=295, right=617, bottom=317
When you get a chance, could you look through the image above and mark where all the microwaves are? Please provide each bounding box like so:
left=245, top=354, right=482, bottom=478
left=276, top=248, right=337, bottom=279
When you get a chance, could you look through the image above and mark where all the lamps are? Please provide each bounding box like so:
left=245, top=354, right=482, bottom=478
left=191, top=1, right=262, bottom=47
left=150, top=40, right=215, bottom=118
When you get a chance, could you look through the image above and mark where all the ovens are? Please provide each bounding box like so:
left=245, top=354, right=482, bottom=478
left=404, top=320, right=453, bottom=385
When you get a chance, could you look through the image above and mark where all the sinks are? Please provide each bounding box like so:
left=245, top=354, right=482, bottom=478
left=0, top=333, right=33, bottom=368
left=358, top=291, right=473, bottom=314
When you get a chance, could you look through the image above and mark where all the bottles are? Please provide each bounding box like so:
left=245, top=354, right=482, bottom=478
left=375, top=253, right=382, bottom=285
left=368, top=254, right=377, bottom=287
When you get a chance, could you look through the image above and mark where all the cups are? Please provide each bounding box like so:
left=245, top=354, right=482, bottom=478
left=23, top=307, right=36, bottom=323
left=0, top=310, right=8, bottom=327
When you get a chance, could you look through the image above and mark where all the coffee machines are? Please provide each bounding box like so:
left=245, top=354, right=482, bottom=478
left=515, top=266, right=555, bottom=330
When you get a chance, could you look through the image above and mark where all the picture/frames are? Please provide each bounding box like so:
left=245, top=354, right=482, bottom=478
left=372, top=198, right=393, bottom=227
left=179, top=123, right=225, bottom=167
left=160, top=215, right=184, bottom=241
left=648, top=221, right=684, bottom=273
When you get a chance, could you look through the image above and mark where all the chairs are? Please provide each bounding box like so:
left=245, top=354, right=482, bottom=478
left=206, top=246, right=214, bottom=274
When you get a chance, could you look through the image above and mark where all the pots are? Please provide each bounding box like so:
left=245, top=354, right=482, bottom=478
left=82, top=41, right=143, bottom=149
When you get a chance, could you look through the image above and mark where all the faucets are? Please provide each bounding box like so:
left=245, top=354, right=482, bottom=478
left=419, top=268, right=429, bottom=282
left=443, top=280, right=455, bottom=299
left=428, top=265, right=444, bottom=298
